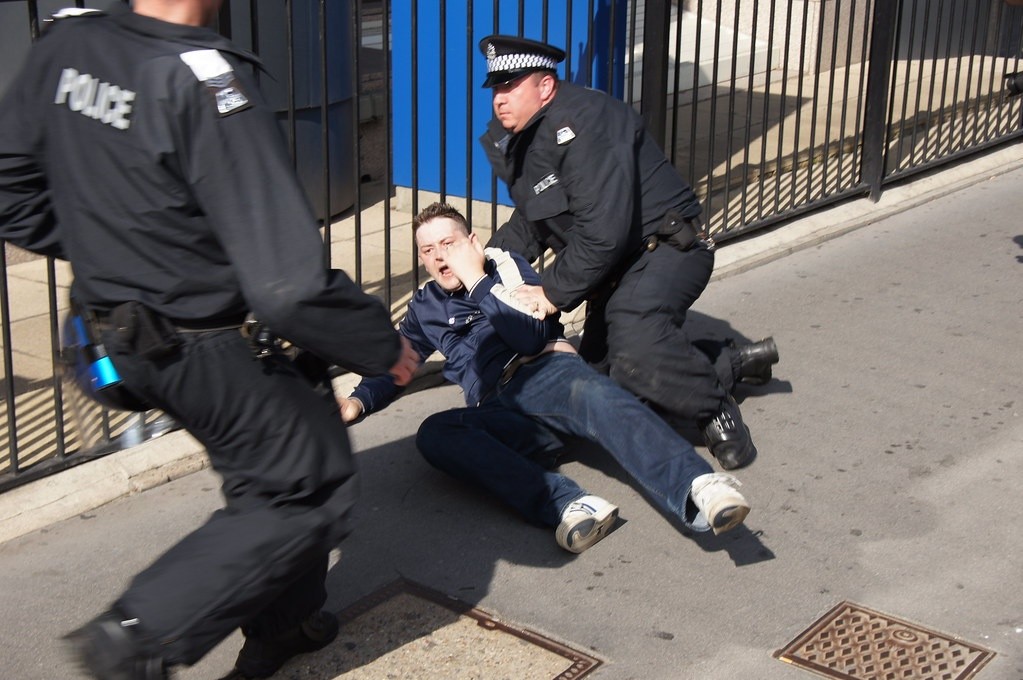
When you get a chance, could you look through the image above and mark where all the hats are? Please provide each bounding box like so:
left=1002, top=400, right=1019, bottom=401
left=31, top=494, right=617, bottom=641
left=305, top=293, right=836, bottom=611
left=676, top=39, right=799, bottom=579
left=479, top=35, right=566, bottom=88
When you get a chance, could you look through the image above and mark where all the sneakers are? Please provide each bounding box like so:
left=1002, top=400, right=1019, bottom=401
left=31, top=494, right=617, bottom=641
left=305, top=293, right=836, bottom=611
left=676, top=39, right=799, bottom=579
left=691, top=472, right=752, bottom=536
left=555, top=495, right=619, bottom=554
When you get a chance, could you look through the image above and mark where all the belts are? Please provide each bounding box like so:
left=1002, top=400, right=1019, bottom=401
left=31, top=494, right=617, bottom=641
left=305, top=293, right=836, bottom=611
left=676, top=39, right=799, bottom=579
left=164, top=305, right=248, bottom=330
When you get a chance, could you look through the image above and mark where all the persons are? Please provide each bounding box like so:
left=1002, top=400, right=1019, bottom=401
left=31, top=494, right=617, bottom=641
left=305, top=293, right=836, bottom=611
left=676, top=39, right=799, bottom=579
left=332, top=202, right=751, bottom=553
left=0, top=0, right=420, bottom=680
left=477, top=35, right=779, bottom=469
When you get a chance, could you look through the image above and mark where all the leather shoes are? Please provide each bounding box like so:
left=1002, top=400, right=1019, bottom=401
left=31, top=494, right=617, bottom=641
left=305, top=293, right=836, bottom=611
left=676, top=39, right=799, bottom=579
left=233, top=610, right=338, bottom=680
left=739, top=336, right=779, bottom=387
left=701, top=394, right=757, bottom=470
left=60, top=605, right=169, bottom=680
